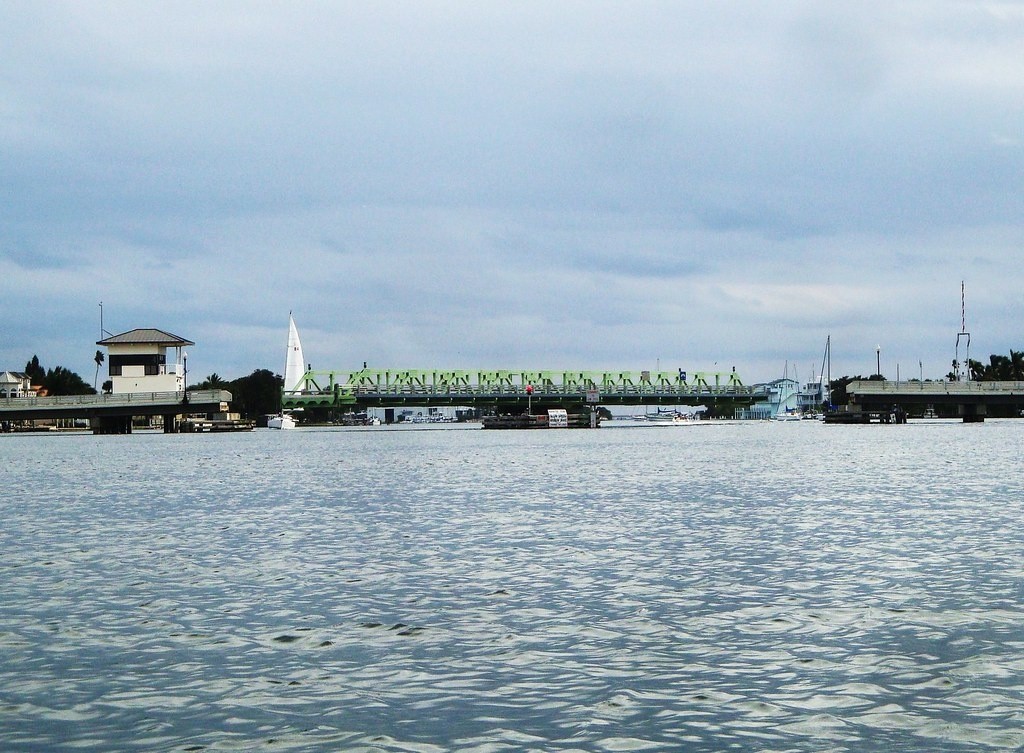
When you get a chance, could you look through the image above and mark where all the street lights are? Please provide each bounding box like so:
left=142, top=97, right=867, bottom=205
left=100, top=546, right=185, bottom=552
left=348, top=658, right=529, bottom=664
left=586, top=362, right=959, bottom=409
left=876, top=345, right=881, bottom=375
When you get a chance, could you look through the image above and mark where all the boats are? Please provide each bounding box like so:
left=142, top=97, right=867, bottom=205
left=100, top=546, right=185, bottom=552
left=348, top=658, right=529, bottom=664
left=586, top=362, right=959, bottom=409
left=267, top=411, right=297, bottom=429
left=633, top=415, right=672, bottom=421
left=480, top=410, right=601, bottom=429
left=774, top=412, right=802, bottom=421
